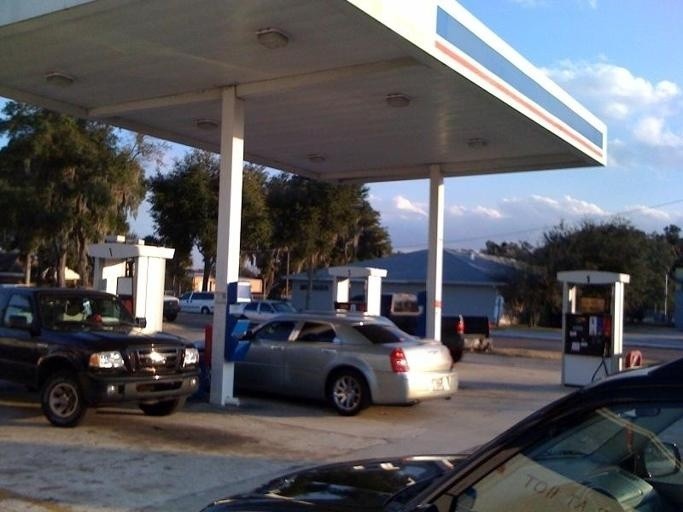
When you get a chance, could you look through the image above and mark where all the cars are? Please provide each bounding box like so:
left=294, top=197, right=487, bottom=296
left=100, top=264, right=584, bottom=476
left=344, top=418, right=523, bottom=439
left=196, top=358, right=682, bottom=512
left=193, top=300, right=457, bottom=415
left=164, top=291, right=216, bottom=321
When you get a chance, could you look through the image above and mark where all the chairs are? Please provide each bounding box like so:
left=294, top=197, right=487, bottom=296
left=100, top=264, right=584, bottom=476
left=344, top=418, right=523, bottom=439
left=59, top=303, right=86, bottom=321
left=87, top=307, right=120, bottom=322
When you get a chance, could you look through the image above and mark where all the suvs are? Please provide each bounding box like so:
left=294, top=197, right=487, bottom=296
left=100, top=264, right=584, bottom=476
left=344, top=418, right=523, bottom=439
left=1, top=285, right=200, bottom=427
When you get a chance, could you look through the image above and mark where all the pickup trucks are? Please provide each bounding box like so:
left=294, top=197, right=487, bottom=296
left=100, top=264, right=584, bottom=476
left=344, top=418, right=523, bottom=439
left=350, top=292, right=491, bottom=363
